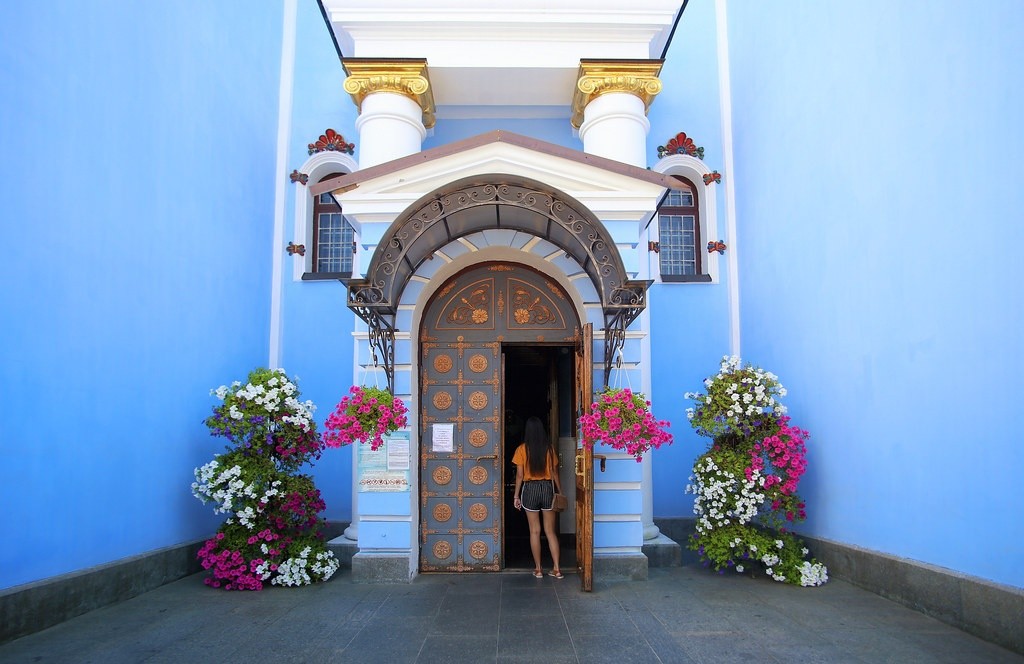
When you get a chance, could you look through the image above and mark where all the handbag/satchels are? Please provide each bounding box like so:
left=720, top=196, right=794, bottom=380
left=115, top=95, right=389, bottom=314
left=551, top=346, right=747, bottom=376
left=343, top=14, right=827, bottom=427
left=552, top=493, right=568, bottom=512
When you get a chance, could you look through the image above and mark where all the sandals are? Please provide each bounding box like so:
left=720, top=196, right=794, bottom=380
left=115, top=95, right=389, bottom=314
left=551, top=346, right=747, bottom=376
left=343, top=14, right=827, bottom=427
left=547, top=569, right=564, bottom=579
left=533, top=569, right=543, bottom=578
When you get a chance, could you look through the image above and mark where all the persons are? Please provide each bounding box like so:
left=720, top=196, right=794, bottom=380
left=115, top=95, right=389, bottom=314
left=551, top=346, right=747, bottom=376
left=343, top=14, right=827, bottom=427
left=512, top=417, right=564, bottom=579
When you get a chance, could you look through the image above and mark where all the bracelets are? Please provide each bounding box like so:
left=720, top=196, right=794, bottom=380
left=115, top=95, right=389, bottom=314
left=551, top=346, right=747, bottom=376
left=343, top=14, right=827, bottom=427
left=514, top=496, right=518, bottom=498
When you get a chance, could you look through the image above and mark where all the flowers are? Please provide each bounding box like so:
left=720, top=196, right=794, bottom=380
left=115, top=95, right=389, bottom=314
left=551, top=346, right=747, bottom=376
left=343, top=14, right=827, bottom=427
left=325, top=383, right=409, bottom=453
left=578, top=387, right=674, bottom=463
left=685, top=352, right=829, bottom=588
left=191, top=368, right=340, bottom=591
